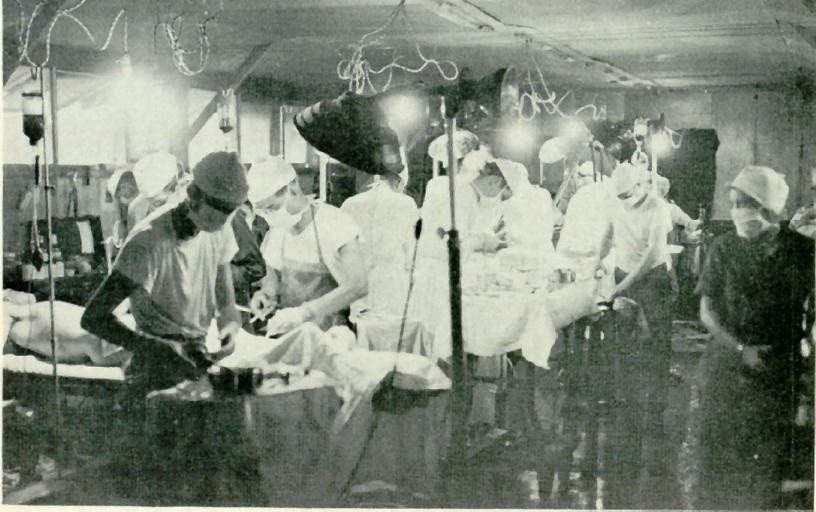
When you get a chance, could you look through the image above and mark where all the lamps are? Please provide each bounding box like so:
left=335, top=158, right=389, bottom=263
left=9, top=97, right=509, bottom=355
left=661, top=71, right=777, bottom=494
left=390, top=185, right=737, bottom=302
left=212, top=85, right=237, bottom=134
left=20, top=68, right=46, bottom=148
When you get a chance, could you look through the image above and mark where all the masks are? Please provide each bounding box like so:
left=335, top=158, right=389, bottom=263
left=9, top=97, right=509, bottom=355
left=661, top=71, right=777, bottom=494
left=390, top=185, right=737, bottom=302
left=186, top=203, right=229, bottom=232
left=731, top=205, right=777, bottom=239
left=263, top=189, right=309, bottom=234
left=475, top=183, right=508, bottom=226
left=618, top=188, right=644, bottom=208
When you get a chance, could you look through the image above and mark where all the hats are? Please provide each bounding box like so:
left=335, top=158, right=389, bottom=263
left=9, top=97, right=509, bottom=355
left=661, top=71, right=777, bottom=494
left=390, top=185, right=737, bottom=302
left=730, top=164, right=789, bottom=214
left=192, top=150, right=248, bottom=204
left=579, top=159, right=597, bottom=177
left=133, top=150, right=177, bottom=198
left=492, top=157, right=529, bottom=196
left=612, top=162, right=638, bottom=196
left=107, top=169, right=126, bottom=198
left=248, top=155, right=296, bottom=201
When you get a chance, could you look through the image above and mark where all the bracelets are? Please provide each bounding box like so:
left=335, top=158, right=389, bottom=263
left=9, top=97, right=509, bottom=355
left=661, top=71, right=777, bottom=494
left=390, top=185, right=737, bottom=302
left=735, top=339, right=746, bottom=364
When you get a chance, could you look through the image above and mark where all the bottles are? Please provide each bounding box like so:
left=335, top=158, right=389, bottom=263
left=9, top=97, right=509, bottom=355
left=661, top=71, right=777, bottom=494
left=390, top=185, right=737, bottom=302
left=40, top=233, right=48, bottom=260
left=53, top=233, right=65, bottom=261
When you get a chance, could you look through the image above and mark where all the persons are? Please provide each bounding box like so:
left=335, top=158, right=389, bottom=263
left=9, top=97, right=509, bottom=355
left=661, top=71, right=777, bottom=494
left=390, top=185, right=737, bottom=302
left=787, top=168, right=816, bottom=243
left=675, top=162, right=816, bottom=508
left=1, top=131, right=707, bottom=510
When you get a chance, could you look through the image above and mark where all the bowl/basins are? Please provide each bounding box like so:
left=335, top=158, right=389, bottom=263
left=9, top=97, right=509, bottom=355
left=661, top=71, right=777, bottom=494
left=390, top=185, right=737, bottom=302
left=208, top=367, right=263, bottom=392
